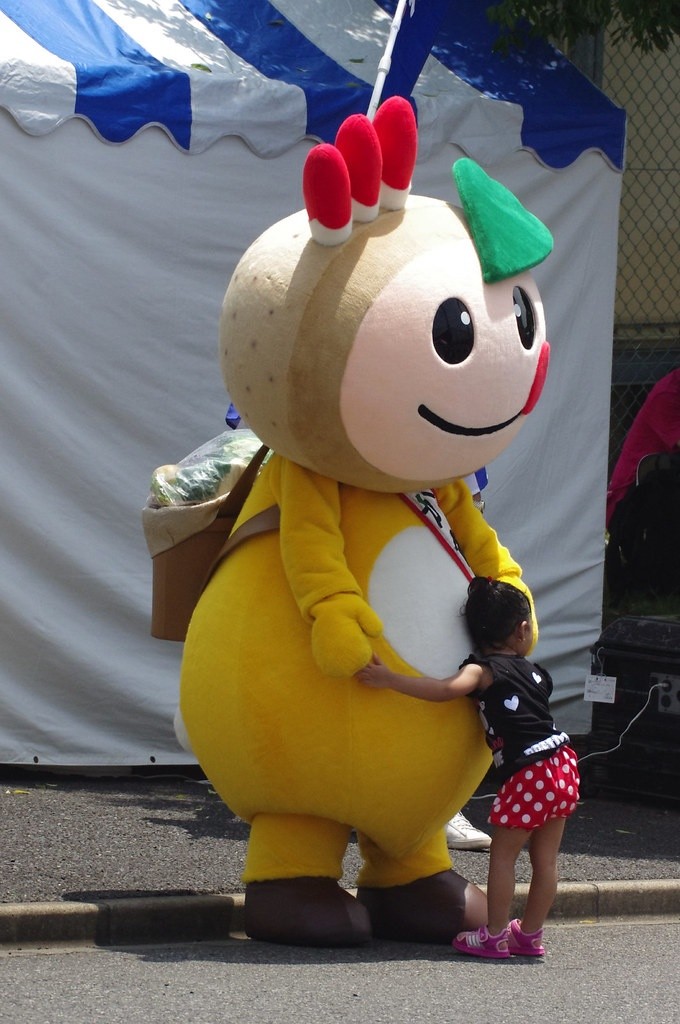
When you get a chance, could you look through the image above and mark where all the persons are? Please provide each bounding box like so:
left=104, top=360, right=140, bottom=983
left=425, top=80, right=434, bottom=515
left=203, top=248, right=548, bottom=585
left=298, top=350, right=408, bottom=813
left=350, top=575, right=582, bottom=950
left=602, top=368, right=680, bottom=597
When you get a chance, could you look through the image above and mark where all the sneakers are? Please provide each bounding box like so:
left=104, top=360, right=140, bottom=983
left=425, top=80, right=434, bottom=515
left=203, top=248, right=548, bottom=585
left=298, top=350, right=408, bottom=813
left=507, top=919, right=545, bottom=956
left=453, top=924, right=510, bottom=958
left=447, top=810, right=492, bottom=849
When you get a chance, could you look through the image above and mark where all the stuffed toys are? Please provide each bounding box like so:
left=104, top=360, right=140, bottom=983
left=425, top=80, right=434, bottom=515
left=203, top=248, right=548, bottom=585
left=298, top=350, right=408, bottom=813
left=184, top=94, right=551, bottom=940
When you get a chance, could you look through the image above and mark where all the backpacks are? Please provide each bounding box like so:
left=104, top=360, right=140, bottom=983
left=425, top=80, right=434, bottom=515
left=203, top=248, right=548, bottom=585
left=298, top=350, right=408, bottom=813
left=607, top=466, right=680, bottom=596
